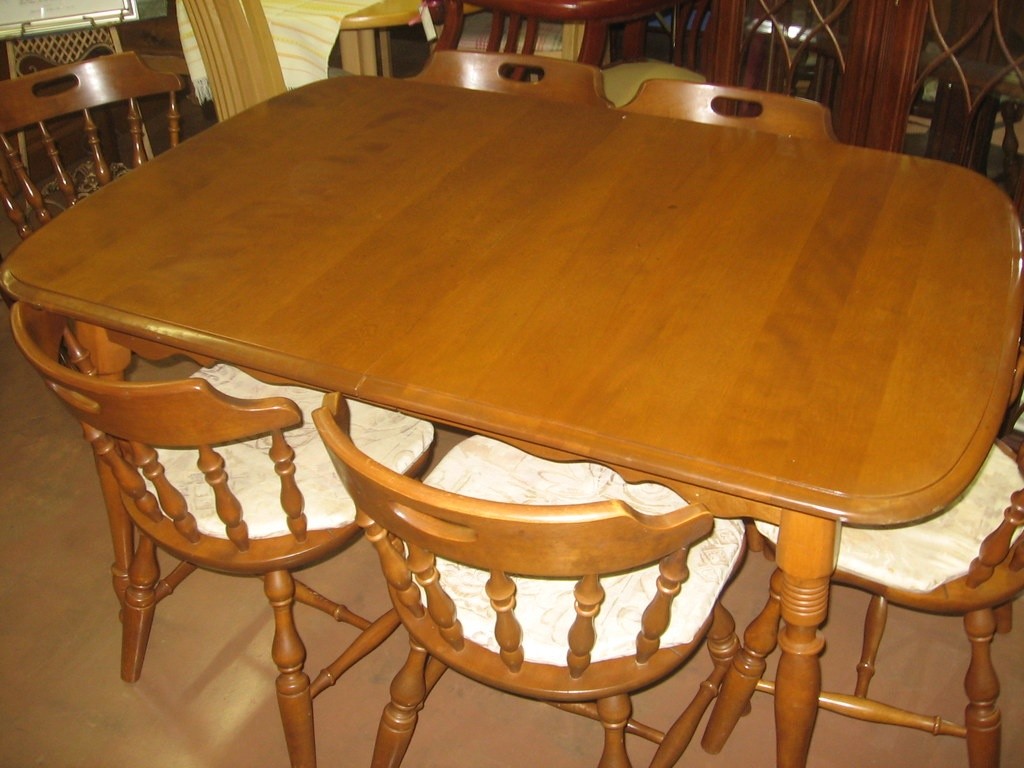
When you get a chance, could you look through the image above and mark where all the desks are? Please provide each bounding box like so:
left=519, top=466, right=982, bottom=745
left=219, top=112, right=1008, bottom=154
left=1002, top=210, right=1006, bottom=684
left=1, top=74, right=1024, bottom=768
left=340, top=0, right=421, bottom=78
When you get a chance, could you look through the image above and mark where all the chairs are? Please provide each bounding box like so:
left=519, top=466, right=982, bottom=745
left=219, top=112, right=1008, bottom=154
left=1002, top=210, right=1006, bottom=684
left=435, top=0, right=673, bottom=67
left=613, top=79, right=838, bottom=143
left=310, top=390, right=746, bottom=768
left=404, top=50, right=614, bottom=108
left=701, top=429, right=1024, bottom=768
left=11, top=300, right=433, bottom=766
left=1, top=51, right=184, bottom=241
